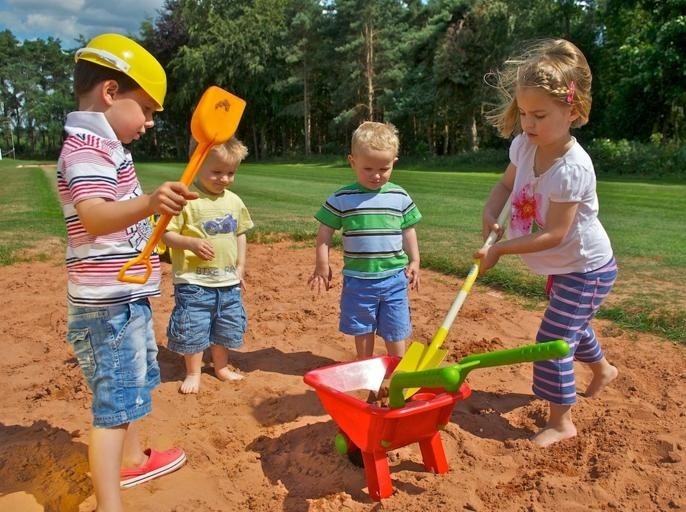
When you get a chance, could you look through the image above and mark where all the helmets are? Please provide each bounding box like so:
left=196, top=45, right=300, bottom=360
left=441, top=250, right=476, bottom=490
left=77, top=32, right=171, bottom=113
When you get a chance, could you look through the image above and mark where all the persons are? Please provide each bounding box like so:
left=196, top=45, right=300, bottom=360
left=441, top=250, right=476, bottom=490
left=471, top=34, right=624, bottom=451
left=162, top=122, right=252, bottom=395
left=51, top=30, right=190, bottom=512
left=302, top=117, right=425, bottom=358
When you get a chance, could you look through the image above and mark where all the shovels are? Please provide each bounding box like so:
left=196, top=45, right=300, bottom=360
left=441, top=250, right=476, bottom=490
left=390, top=196, right=512, bottom=400
left=117, top=86, right=249, bottom=286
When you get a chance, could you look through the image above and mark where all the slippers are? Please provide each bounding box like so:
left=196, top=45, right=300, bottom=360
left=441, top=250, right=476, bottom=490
left=119, top=446, right=187, bottom=491
